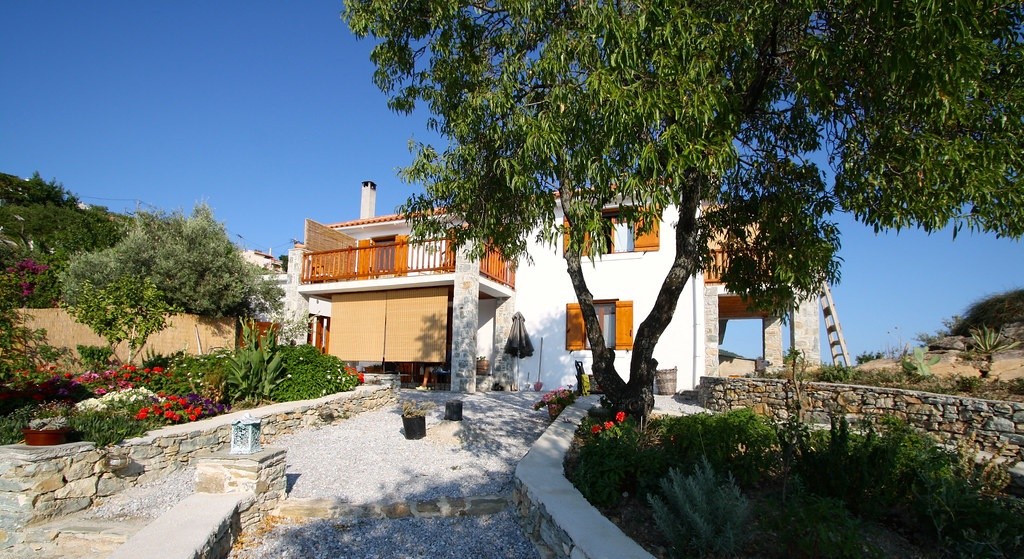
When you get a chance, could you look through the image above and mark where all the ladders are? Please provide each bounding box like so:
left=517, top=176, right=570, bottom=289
left=821, top=273, right=852, bottom=369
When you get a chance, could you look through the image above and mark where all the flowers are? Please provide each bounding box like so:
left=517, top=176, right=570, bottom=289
left=533, top=384, right=574, bottom=411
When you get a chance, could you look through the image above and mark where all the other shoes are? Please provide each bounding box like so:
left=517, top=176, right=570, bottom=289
left=415, top=386, right=428, bottom=392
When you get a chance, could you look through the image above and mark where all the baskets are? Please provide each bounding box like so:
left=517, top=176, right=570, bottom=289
left=655, top=366, right=678, bottom=395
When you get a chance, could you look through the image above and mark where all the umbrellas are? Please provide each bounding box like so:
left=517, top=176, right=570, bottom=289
left=503, top=311, right=534, bottom=390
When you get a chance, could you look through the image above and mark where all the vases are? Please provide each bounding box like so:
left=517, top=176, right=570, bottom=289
left=548, top=409, right=559, bottom=422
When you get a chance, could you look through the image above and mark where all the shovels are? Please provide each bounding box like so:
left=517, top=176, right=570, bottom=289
left=523, top=372, right=531, bottom=392
left=534, top=337, right=543, bottom=391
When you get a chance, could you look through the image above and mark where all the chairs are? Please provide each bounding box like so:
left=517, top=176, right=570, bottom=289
left=430, top=360, right=451, bottom=391
left=381, top=361, right=412, bottom=389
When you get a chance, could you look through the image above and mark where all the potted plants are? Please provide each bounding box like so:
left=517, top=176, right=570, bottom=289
left=20, top=415, right=70, bottom=446
left=401, top=399, right=438, bottom=440
left=476, top=356, right=490, bottom=375
left=288, top=238, right=305, bottom=249
